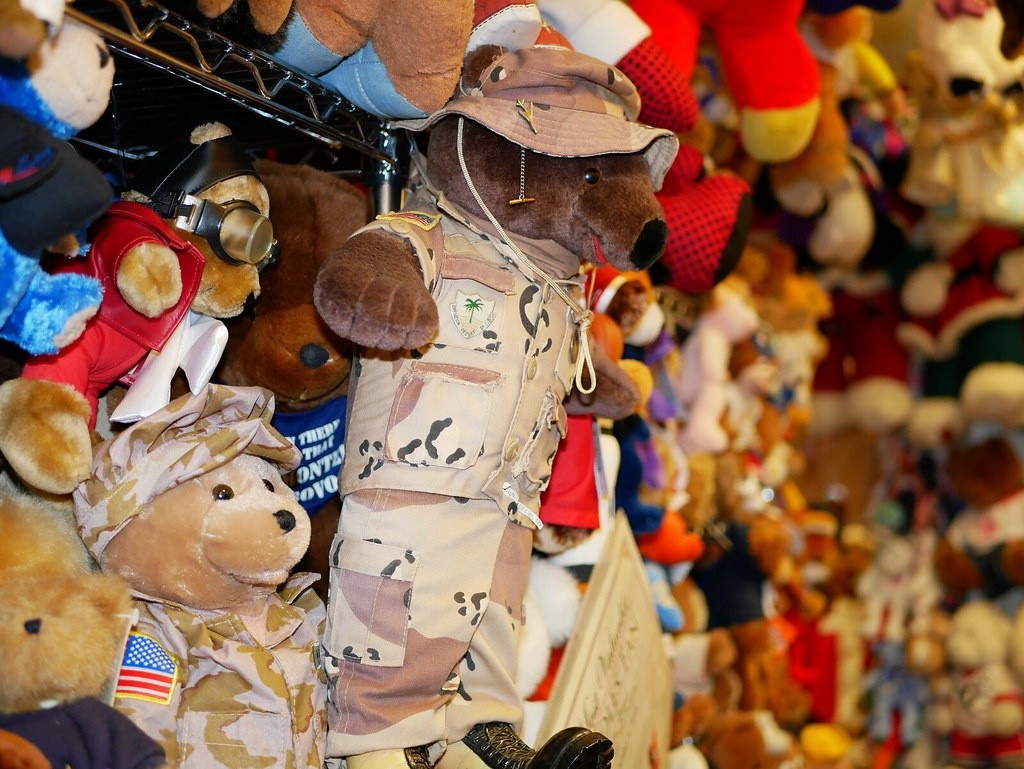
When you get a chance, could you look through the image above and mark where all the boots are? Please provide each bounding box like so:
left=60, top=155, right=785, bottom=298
left=461, top=721, right=614, bottom=769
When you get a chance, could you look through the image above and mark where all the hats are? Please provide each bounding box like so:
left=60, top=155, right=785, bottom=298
left=391, top=46, right=679, bottom=193
left=71, top=385, right=302, bottom=566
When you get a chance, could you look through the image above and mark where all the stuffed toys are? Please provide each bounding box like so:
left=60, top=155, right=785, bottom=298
left=0, top=0, right=1024, bottom=769
